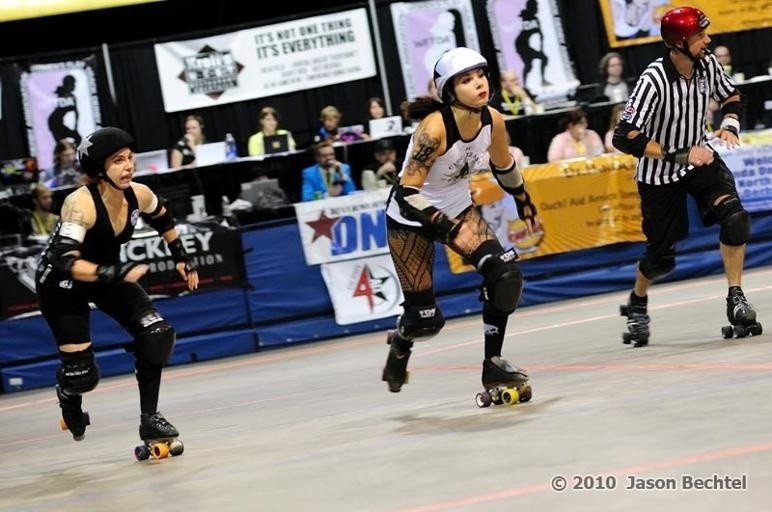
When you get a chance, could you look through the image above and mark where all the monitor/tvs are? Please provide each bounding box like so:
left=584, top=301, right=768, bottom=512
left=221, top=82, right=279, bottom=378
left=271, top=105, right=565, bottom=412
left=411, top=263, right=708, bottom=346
left=370, top=116, right=403, bottom=138
left=240, top=179, right=279, bottom=206
left=194, top=141, right=227, bottom=163
left=134, top=150, right=170, bottom=171
left=263, top=134, right=290, bottom=153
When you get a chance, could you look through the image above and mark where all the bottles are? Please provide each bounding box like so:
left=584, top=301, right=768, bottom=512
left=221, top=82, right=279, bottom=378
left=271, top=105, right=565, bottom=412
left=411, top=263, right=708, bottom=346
left=191, top=194, right=207, bottom=220
left=361, top=169, right=388, bottom=191
left=315, top=190, right=329, bottom=200
left=224, top=133, right=237, bottom=162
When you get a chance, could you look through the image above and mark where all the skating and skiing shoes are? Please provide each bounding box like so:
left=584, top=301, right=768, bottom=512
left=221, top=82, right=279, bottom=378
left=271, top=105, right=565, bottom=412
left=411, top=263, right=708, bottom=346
left=135, top=412, right=184, bottom=460
left=56, top=384, right=90, bottom=440
left=476, top=355, right=532, bottom=406
left=382, top=325, right=413, bottom=394
left=721, top=287, right=762, bottom=338
left=620, top=293, right=650, bottom=347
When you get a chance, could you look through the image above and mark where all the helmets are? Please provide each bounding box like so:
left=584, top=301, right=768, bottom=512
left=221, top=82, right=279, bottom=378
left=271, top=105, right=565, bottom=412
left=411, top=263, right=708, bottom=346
left=660, top=7, right=709, bottom=48
left=79, top=127, right=133, bottom=177
left=433, top=46, right=487, bottom=101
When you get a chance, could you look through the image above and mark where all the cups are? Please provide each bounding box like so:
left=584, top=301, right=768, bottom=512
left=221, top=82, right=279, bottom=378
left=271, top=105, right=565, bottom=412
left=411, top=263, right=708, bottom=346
left=721, top=64, right=745, bottom=86
left=523, top=100, right=544, bottom=116
left=519, top=156, right=530, bottom=166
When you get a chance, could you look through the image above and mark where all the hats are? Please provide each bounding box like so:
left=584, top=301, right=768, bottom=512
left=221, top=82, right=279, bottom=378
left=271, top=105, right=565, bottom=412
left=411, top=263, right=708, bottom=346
left=376, top=139, right=395, bottom=152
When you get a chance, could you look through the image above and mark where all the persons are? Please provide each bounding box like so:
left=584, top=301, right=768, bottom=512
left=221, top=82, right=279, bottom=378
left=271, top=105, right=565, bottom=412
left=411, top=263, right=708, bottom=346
left=613, top=6, right=756, bottom=341
left=0, top=44, right=771, bottom=238
left=384, top=47, right=538, bottom=391
left=35, top=126, right=201, bottom=442
